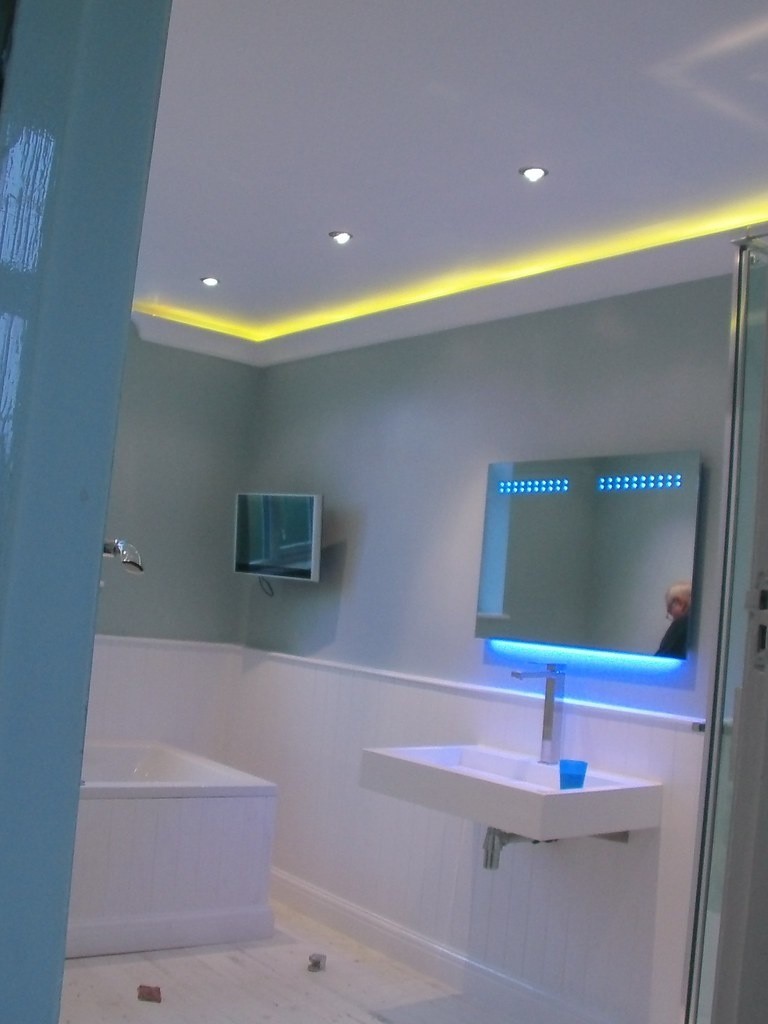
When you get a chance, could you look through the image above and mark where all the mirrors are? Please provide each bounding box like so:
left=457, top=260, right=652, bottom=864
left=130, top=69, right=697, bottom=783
left=473, top=448, right=704, bottom=662
left=232, top=491, right=323, bottom=584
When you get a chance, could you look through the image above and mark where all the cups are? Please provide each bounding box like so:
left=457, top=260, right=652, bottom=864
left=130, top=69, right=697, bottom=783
left=558, top=758, right=588, bottom=794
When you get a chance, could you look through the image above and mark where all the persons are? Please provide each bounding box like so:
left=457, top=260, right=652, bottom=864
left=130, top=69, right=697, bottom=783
left=654, top=581, right=691, bottom=660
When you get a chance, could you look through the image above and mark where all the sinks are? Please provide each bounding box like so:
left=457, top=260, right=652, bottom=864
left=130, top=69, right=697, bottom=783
left=357, top=742, right=663, bottom=842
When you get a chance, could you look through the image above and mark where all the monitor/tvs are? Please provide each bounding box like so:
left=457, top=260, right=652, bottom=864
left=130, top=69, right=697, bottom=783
left=233, top=492, right=322, bottom=586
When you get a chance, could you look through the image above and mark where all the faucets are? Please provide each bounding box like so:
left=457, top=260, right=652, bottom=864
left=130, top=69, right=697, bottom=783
left=509, top=656, right=570, bottom=766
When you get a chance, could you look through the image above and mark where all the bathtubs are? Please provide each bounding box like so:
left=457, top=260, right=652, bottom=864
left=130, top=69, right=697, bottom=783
left=60, top=738, right=280, bottom=961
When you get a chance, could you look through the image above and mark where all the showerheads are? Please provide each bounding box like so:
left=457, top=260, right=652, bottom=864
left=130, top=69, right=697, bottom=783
left=102, top=538, right=145, bottom=576
